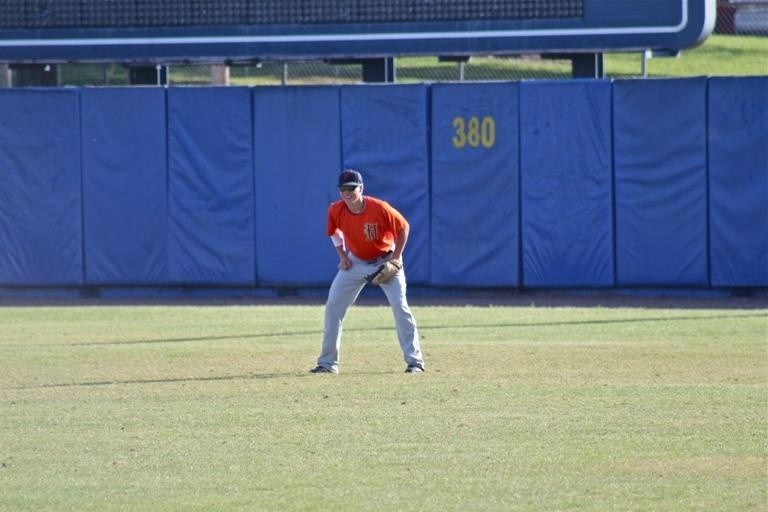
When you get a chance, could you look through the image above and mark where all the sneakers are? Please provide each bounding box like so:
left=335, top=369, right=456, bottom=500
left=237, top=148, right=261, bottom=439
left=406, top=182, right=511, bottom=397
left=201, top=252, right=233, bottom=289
left=405, top=364, right=423, bottom=372
left=310, top=366, right=338, bottom=373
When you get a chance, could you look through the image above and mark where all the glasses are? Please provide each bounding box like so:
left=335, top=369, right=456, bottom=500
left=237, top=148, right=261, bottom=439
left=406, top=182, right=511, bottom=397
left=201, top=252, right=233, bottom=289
left=338, top=186, right=358, bottom=192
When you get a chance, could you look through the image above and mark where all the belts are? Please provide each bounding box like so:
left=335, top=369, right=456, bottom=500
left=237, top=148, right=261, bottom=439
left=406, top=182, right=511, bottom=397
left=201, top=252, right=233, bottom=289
left=368, top=249, right=393, bottom=264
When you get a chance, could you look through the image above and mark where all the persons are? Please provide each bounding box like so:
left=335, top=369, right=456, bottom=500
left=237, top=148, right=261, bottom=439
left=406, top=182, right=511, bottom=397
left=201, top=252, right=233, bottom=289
left=308, top=168, right=427, bottom=375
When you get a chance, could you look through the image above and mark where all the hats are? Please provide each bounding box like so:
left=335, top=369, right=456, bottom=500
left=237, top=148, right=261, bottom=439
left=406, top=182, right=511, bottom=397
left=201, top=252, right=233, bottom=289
left=336, top=170, right=364, bottom=186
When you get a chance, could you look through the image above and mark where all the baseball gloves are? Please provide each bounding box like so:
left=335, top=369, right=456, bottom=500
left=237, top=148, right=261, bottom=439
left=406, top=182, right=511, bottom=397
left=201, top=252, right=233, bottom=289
left=362, top=260, right=402, bottom=285
